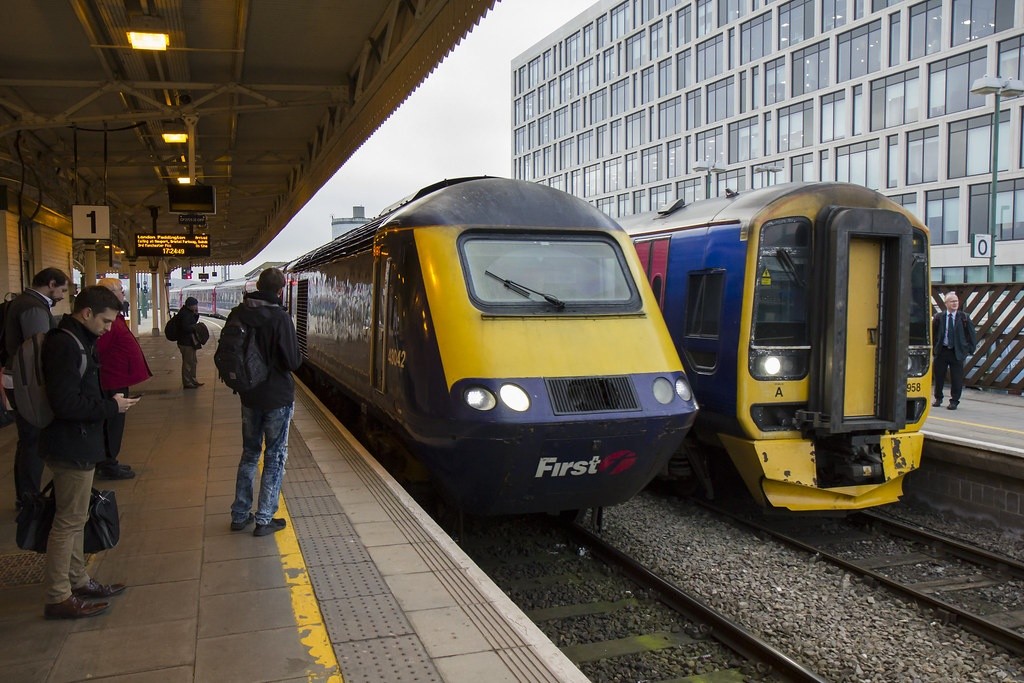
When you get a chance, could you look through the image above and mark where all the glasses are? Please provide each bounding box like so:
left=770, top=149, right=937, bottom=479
left=114, top=290, right=125, bottom=294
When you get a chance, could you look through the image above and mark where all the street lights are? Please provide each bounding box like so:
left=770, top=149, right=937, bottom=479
left=970, top=74, right=1024, bottom=390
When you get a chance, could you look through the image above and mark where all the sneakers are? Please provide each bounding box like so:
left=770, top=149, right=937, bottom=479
left=231, top=512, right=254, bottom=529
left=115, top=465, right=131, bottom=470
left=254, top=519, right=286, bottom=536
left=100, top=465, right=135, bottom=479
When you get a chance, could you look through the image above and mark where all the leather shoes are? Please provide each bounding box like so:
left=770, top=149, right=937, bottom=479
left=46, top=596, right=110, bottom=617
left=71, top=579, right=125, bottom=596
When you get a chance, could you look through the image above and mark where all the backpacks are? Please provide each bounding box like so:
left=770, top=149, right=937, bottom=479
left=13, top=328, right=87, bottom=428
left=0, top=292, right=19, bottom=368
left=214, top=312, right=268, bottom=394
left=165, top=307, right=189, bottom=341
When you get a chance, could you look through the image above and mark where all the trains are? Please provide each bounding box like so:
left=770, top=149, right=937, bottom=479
left=614, top=181, right=938, bottom=521
left=170, top=174, right=703, bottom=520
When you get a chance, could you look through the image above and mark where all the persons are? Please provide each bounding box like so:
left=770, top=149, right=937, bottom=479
left=0, top=268, right=71, bottom=511
left=96, top=277, right=152, bottom=480
left=933, top=294, right=976, bottom=410
left=40, top=284, right=141, bottom=619
left=122, top=298, right=129, bottom=316
left=215, top=268, right=300, bottom=537
left=177, top=297, right=206, bottom=390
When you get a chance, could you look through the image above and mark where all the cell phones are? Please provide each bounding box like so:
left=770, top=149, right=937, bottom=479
left=130, top=392, right=145, bottom=399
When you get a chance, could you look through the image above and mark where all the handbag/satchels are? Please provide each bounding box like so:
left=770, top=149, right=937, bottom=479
left=15, top=479, right=120, bottom=552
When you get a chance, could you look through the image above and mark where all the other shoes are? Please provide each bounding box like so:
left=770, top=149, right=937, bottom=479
left=194, top=382, right=204, bottom=386
left=185, top=384, right=197, bottom=389
